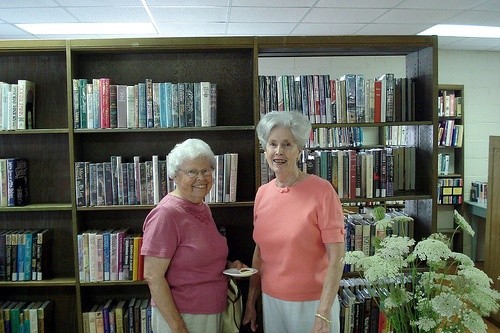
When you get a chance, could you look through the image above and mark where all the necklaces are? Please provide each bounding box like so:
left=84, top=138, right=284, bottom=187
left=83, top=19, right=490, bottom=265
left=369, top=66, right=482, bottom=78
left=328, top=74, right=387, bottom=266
left=275, top=169, right=301, bottom=193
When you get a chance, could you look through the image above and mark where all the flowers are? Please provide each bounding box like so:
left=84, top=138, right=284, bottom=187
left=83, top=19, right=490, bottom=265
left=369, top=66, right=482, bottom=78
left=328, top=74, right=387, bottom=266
left=339, top=206, right=500, bottom=333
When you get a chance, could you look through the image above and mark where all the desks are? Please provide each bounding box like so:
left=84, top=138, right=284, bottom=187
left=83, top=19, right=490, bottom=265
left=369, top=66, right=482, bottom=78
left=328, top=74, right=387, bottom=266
left=465, top=200, right=487, bottom=264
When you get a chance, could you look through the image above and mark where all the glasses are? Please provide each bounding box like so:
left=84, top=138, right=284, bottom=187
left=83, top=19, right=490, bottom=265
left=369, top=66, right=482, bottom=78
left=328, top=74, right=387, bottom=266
left=178, top=166, right=214, bottom=178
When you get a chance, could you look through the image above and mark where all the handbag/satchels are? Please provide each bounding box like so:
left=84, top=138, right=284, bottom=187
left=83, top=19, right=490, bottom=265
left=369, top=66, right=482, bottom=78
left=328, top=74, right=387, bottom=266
left=223, top=276, right=244, bottom=333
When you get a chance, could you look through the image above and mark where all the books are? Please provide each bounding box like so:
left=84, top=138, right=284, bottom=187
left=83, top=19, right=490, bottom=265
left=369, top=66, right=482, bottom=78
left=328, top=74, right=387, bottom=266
left=72, top=79, right=239, bottom=333
left=0, top=79, right=53, bottom=333
left=437, top=90, right=464, bottom=205
left=257, top=73, right=416, bottom=333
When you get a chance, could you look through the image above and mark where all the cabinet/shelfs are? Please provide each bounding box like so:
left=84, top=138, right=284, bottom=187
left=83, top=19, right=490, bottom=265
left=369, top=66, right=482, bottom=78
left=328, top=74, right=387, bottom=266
left=438, top=84, right=465, bottom=253
left=0, top=35, right=439, bottom=333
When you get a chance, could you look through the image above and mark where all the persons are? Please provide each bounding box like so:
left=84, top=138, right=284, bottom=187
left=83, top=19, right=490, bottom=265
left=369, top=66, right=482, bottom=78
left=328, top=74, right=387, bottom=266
left=242, top=110, right=346, bottom=333
left=140, top=138, right=249, bottom=333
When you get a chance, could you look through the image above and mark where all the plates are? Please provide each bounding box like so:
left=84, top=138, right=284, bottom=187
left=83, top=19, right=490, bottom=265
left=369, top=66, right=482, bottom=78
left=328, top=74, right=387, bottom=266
left=223, top=268, right=259, bottom=277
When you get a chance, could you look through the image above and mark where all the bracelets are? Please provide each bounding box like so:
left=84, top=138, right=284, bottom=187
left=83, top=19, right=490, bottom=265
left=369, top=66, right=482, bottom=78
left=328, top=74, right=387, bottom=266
left=315, top=313, right=332, bottom=325
